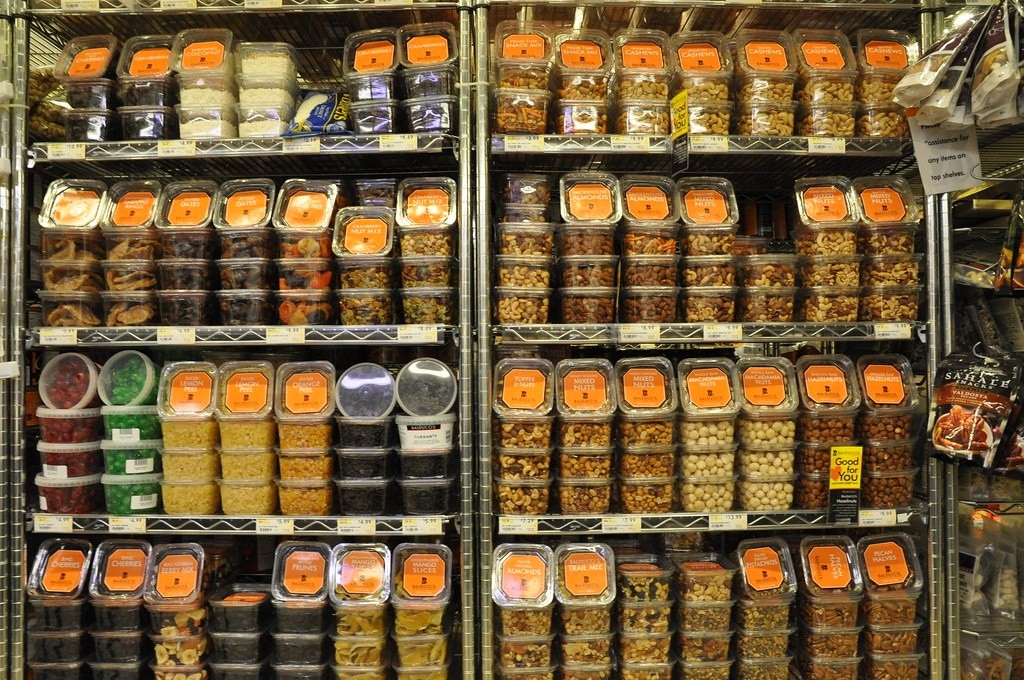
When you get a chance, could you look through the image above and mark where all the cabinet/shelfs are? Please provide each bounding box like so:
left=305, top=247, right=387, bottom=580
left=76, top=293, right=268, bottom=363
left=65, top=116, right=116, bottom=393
left=0, top=0, right=1024, bottom=680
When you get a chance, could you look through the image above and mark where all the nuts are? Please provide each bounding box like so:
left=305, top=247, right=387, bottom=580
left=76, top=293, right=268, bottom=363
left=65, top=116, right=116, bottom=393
left=497, top=183, right=922, bottom=330
left=496, top=29, right=910, bottom=141
left=495, top=532, right=921, bottom=680
left=499, top=411, right=918, bottom=513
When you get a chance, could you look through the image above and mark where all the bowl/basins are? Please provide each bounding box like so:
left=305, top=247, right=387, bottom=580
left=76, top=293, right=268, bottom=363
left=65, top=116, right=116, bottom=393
left=24, top=539, right=455, bottom=680
left=492, top=345, right=921, bottom=515
left=51, top=21, right=459, bottom=142
left=491, top=532, right=925, bottom=680
left=34, top=350, right=458, bottom=515
left=37, top=177, right=457, bottom=327
left=493, top=173, right=925, bottom=325
left=492, top=21, right=921, bottom=138
